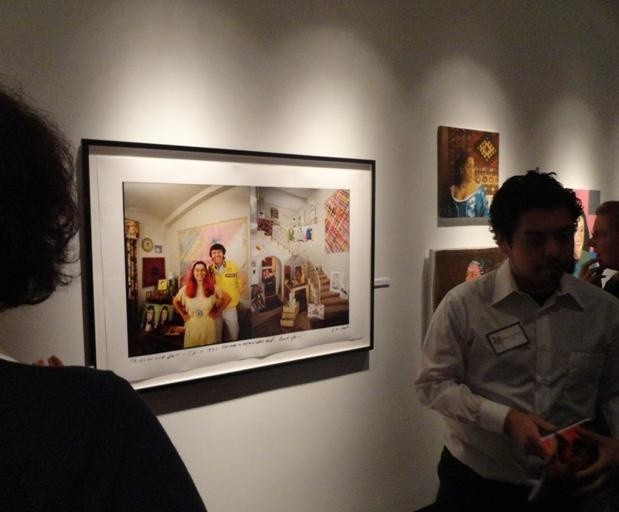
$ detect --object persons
[140,305,156,332]
[156,306,170,328]
[208,243,242,342]
[0,82,210,511]
[464,257,489,282]
[573,212,597,278]
[172,260,232,348]
[440,152,489,217]
[578,200,619,299]
[416,166,619,512]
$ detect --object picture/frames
[82,138,376,392]
